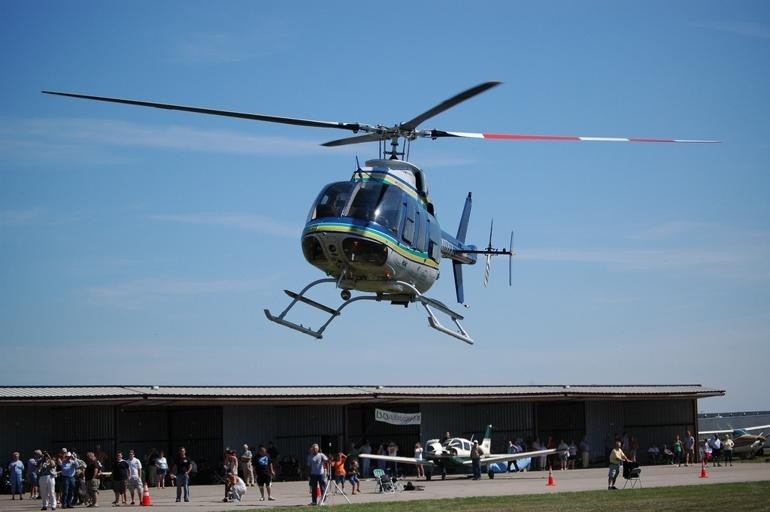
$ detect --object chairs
[373,469,405,494]
[623,462,643,488]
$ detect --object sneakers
[19,497,23,500]
[222,498,228,502]
[268,497,275,500]
[308,502,317,506]
[334,489,361,496]
[29,495,38,499]
[175,497,181,502]
[472,476,481,480]
[505,466,575,474]
[259,497,265,501]
[41,507,47,511]
[671,463,734,468]
[184,497,189,502]
[62,502,97,509]
[51,507,56,511]
[112,500,135,507]
[11,496,15,500]
[607,485,618,490]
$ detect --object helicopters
[38,81,727,349]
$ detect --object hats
[474,440,479,444]
[34,449,44,456]
[62,447,67,453]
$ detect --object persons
[8,441,299,510]
[332,442,400,494]
[507,431,733,471]
[306,443,330,505]
[608,440,632,489]
[471,439,484,480]
[414,442,425,478]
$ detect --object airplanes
[355,425,566,480]
[697,418,770,460]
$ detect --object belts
[609,463,619,466]
[63,476,74,479]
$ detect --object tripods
[318,458,352,507]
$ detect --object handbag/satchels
[258,456,268,465]
[49,468,57,477]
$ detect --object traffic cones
[698,460,710,479]
[316,480,323,497]
[546,465,558,486]
[138,481,154,508]
